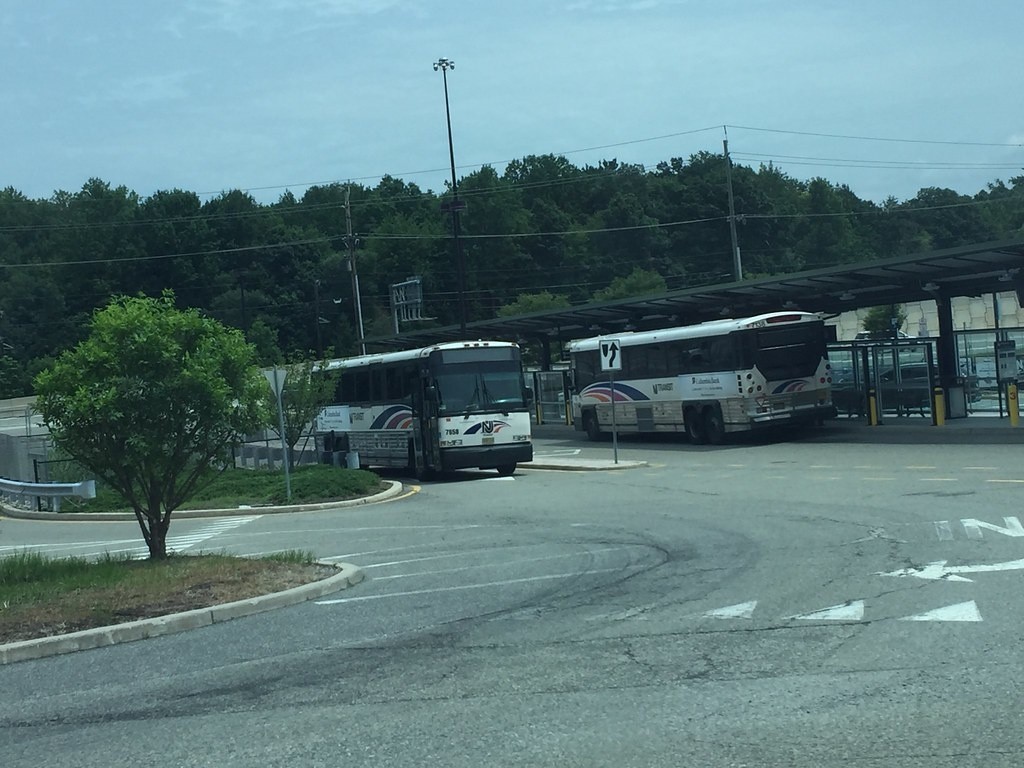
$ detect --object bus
[311,341,534,482]
[566,311,837,447]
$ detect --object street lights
[433,57,461,205]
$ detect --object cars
[869,362,982,409]
